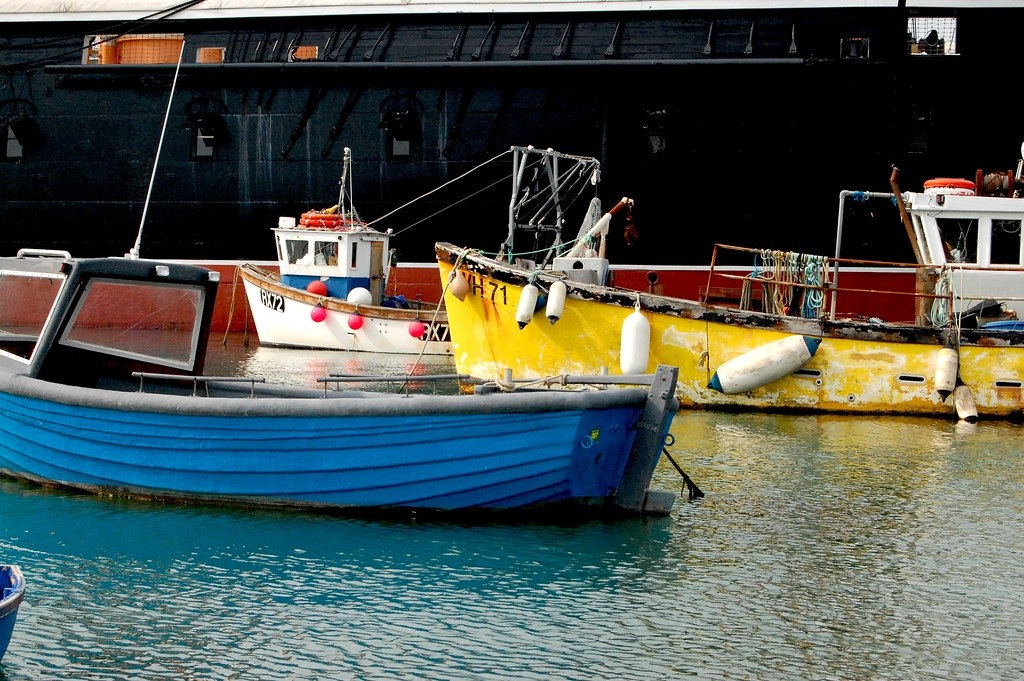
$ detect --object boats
[0,40,682,518]
[235,146,458,354]
[430,164,1023,421]
[0,562,27,663]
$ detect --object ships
[0,5,1023,266]
